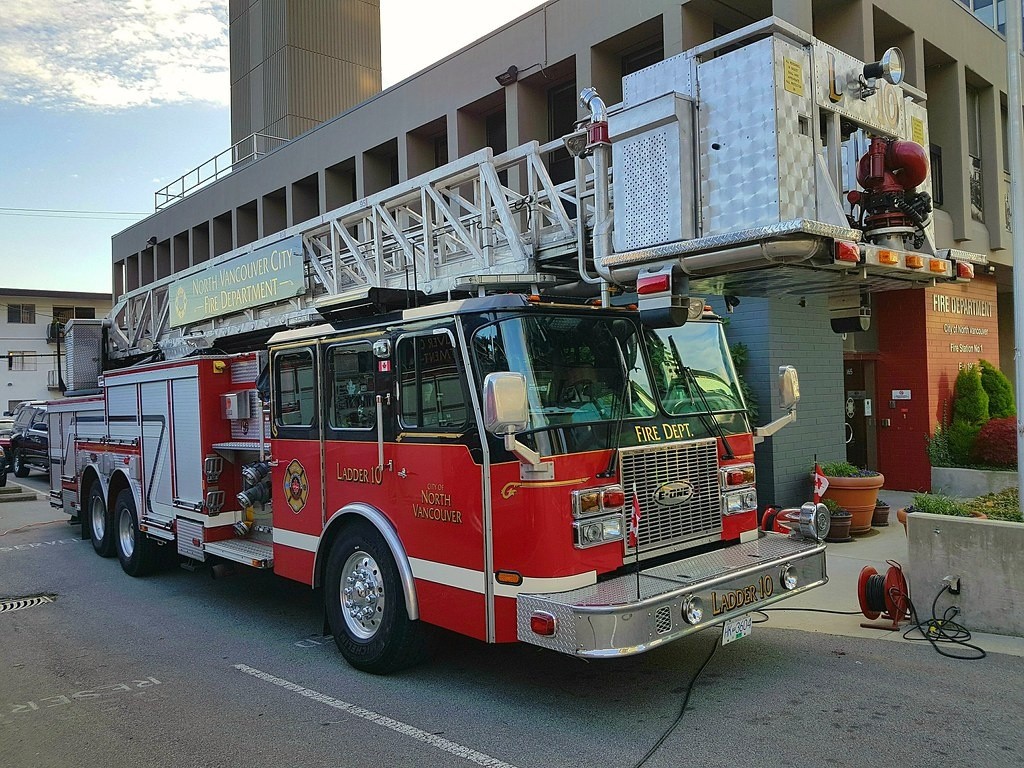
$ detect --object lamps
[724,294,739,309]
[857,47,906,100]
[494,65,518,87]
[146,236,157,245]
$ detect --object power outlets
[726,306,733,313]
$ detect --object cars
[0,400,46,487]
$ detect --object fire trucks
[47,13,974,687]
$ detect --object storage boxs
[220,389,250,422]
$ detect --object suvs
[12,401,49,479]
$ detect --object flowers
[851,469,879,478]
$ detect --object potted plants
[871,499,890,527]
[809,462,885,534]
[825,499,852,541]
[897,487,986,538]
